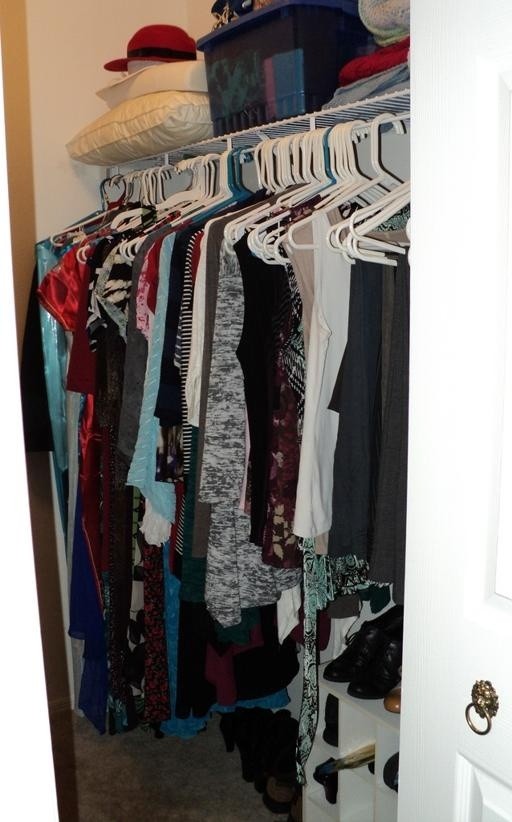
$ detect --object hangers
[45,115,410,273]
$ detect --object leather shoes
[324,604,403,713]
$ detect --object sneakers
[219,706,300,815]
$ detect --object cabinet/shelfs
[301,661,399,820]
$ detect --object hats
[103,25,199,73]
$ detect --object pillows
[67,90,213,165]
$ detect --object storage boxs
[194,2,369,133]
